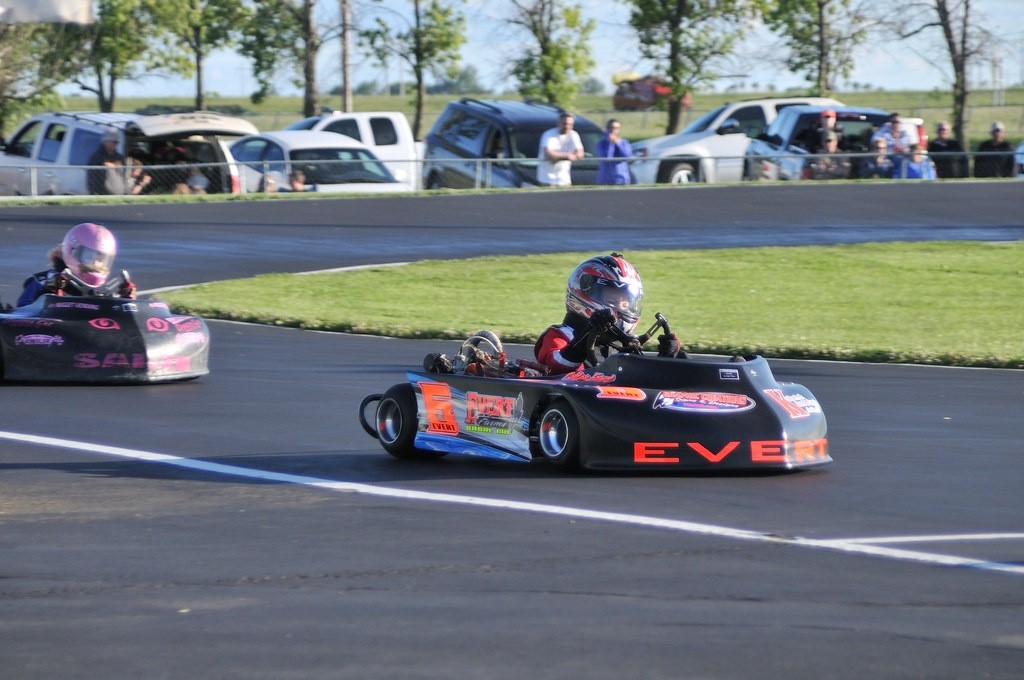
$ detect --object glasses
[891,120,902,124]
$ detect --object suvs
[0,103,260,197]
[630,96,847,184]
[741,104,892,183]
[422,97,607,189]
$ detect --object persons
[742,109,1019,182]
[537,112,584,188]
[15,223,135,309]
[534,252,682,377]
[595,118,634,185]
[85,132,310,197]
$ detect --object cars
[225,130,412,193]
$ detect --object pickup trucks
[280,105,426,190]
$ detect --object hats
[821,130,837,144]
[98,126,121,143]
[937,121,950,131]
[821,109,837,118]
[990,121,1003,132]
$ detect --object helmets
[61,223,117,289]
[564,252,643,336]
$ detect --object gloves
[587,307,616,332]
[44,270,71,295]
[117,281,137,299]
[657,333,682,359]
